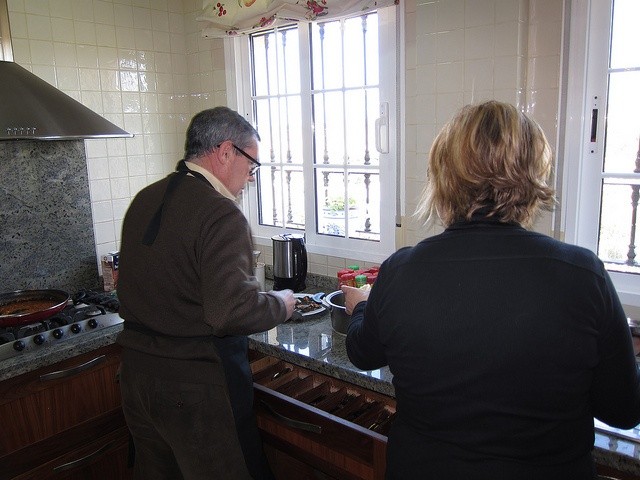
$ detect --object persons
[114,106,298,479]
[340,101,640,480]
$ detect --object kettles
[271,234,308,292]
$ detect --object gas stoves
[0,288,126,360]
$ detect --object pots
[0,288,70,324]
[326,289,360,337]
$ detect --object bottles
[254,250,266,295]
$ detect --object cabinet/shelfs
[0,340,136,479]
[591,464,639,480]
[247,347,397,479]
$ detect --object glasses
[217,144,261,175]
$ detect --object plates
[292,293,327,317]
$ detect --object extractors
[0,0,136,141]
